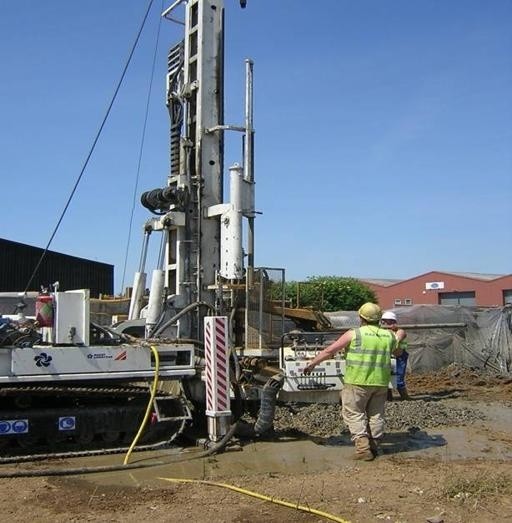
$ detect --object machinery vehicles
[0,0,398,463]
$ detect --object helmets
[358,302,382,322]
[380,311,397,323]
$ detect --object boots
[398,387,411,401]
[369,438,384,455]
[387,389,393,402]
[348,435,373,460]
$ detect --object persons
[301,301,400,461]
[380,310,409,401]
